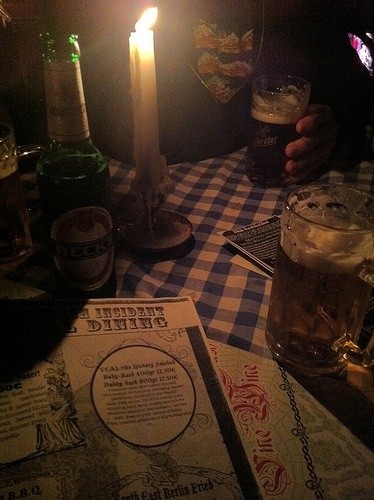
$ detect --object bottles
[36,32,118,308]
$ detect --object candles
[128,7,161,178]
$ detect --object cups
[245,73,310,187]
[264,185,374,376]
[0,125,33,274]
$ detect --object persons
[46,0,374,185]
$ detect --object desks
[0,146,374,451]
